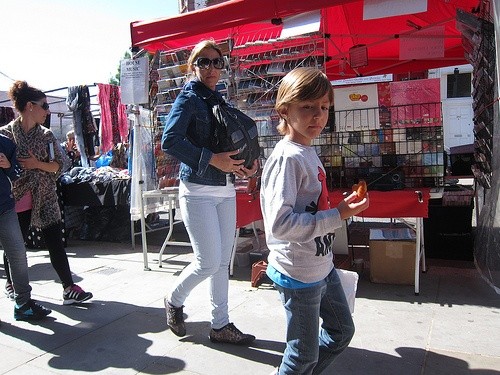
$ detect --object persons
[259,67,370,375]
[0,134,52,321]
[59,129,130,170]
[160,40,260,346]
[0,81,93,306]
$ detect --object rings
[231,169,233,172]
[23,164,24,167]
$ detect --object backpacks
[183,87,260,174]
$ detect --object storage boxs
[369,227,417,286]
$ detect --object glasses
[30,100,50,110]
[192,57,224,69]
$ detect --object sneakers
[4,281,16,301]
[209,323,255,345]
[163,295,187,337]
[62,284,93,304]
[13,299,52,319]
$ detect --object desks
[159,188,430,295]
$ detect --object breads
[352,180,367,203]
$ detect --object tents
[130,0,495,272]
